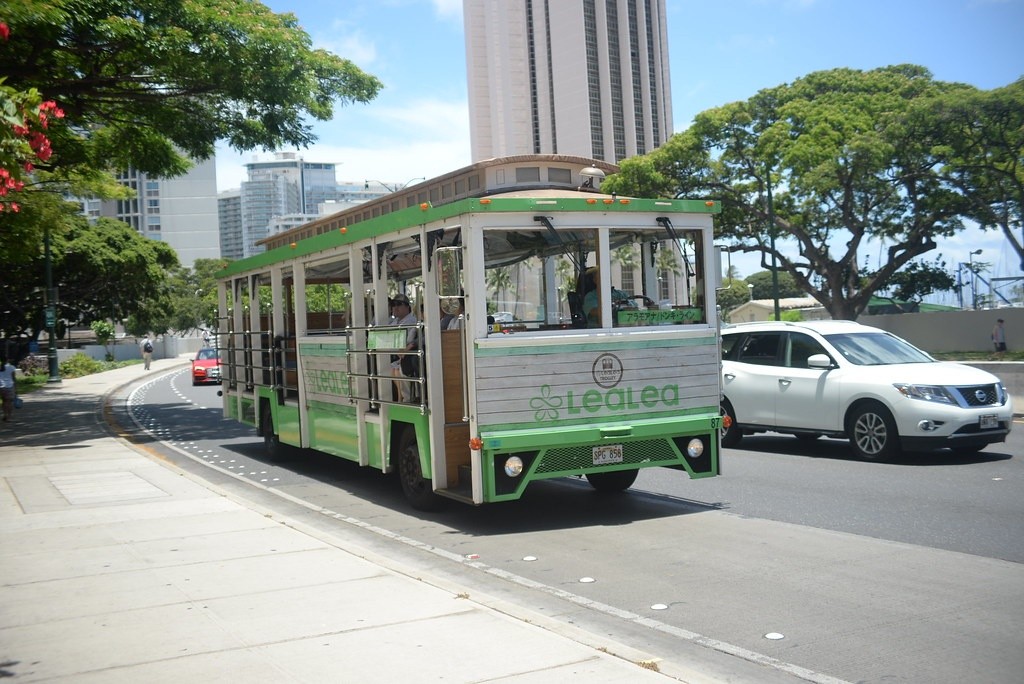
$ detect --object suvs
[721,319,1012,462]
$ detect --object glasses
[391,302,405,307]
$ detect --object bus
[212,153,731,511]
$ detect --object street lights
[970,249,982,309]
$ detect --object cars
[189,349,222,386]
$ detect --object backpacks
[144,339,153,353]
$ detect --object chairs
[567,267,596,329]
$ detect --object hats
[391,294,409,304]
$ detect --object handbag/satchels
[401,355,414,376]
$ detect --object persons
[367,289,465,404]
[140,335,152,369]
[202,328,210,348]
[584,268,637,327]
[990,319,1006,361]
[0,355,18,422]
